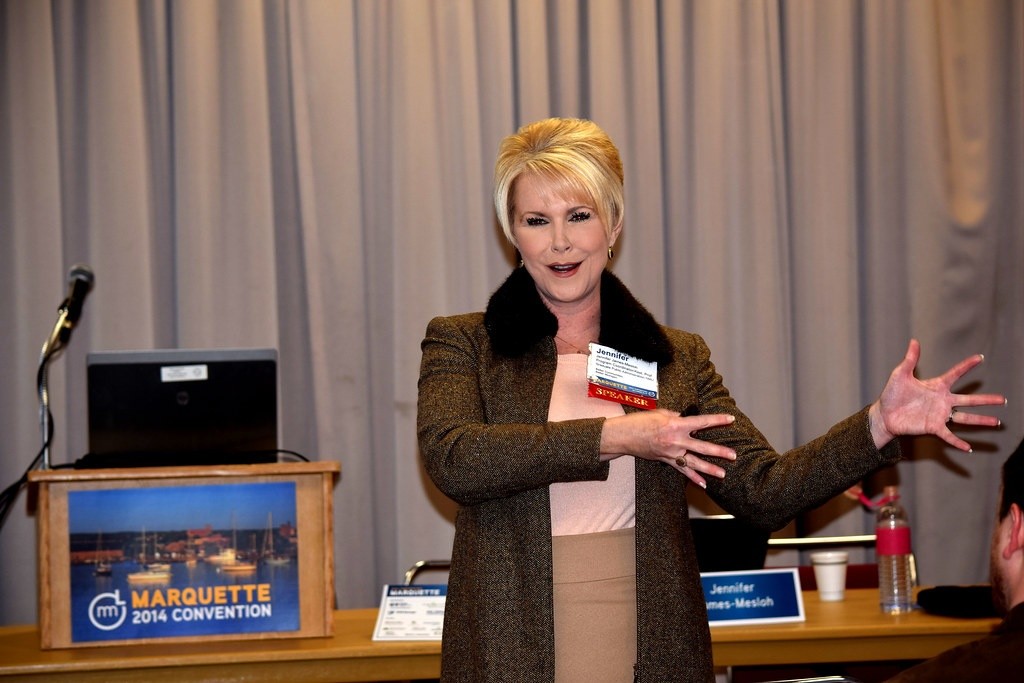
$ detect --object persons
[414,114,1008,683]
[876,436,1024,683]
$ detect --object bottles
[878,486,912,615]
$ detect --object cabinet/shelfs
[26,461,344,649]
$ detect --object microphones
[54,262,96,346]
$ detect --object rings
[676,450,689,467]
[949,408,959,423]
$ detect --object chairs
[405,515,767,587]
[768,535,913,589]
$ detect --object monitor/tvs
[83,347,279,465]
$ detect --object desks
[1,581,1005,682]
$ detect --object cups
[810,550,847,601]
